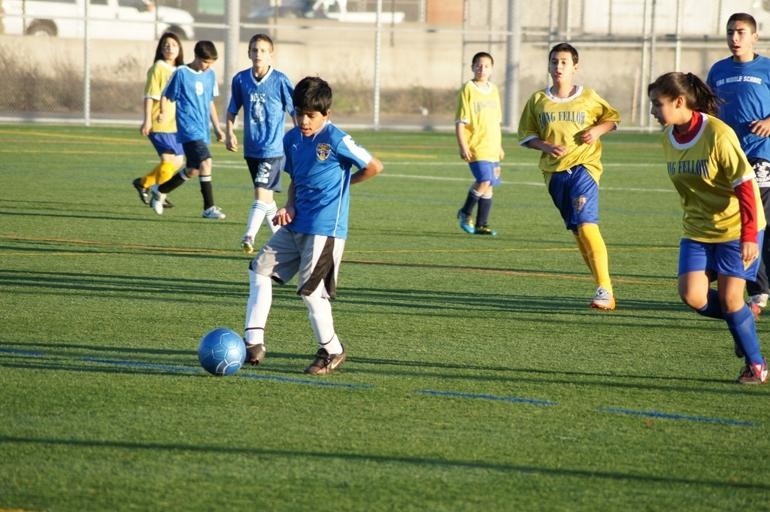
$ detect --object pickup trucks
[305,0,406,23]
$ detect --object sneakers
[150,184,166,216]
[240,235,255,254]
[202,206,225,219]
[747,294,769,313]
[163,199,174,208]
[241,337,266,365]
[735,302,759,358]
[737,357,769,385]
[456,208,474,234]
[132,178,149,204]
[304,343,346,377]
[474,224,496,236]
[591,286,616,311]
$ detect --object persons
[516,41,622,310]
[224,33,297,256]
[133,31,185,208]
[454,51,506,236]
[706,12,770,314]
[647,71,769,384]
[150,39,227,220]
[242,75,384,374]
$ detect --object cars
[254,5,302,22]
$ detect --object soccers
[197,327,247,377]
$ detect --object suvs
[1,0,197,42]
